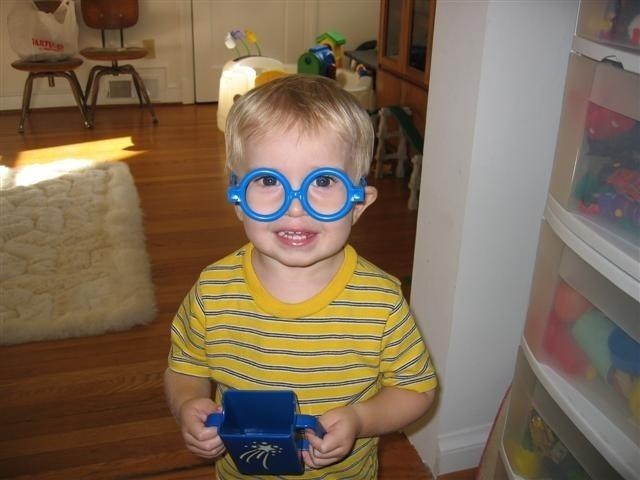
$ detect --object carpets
[0,158,160,345]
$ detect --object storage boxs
[478,346,623,480]
[540,45,639,285]
[517,203,640,478]
[573,0,638,73]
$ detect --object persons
[161,73,438,480]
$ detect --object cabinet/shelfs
[371,0,436,167]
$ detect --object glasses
[226,168,368,221]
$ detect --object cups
[204,389,325,476]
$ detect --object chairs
[9,1,160,135]
